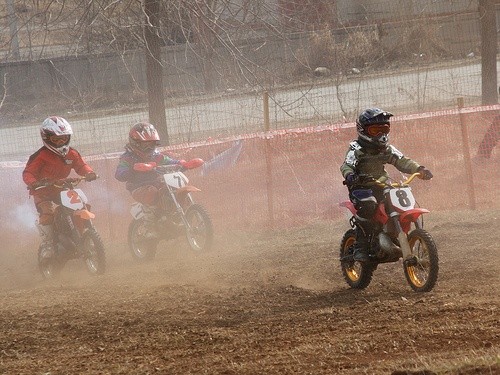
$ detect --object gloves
[418,166,433,180]
[85,171,96,182]
[347,173,360,188]
[31,181,43,191]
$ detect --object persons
[115,123,205,233]
[341,107,433,263]
[22,114,99,264]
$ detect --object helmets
[40,116,71,157]
[356,108,394,146]
[126,122,160,159]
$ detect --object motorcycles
[127,158,214,261]
[339,172,439,293]
[37,175,107,281]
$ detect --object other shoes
[145,231,160,239]
[354,248,369,261]
